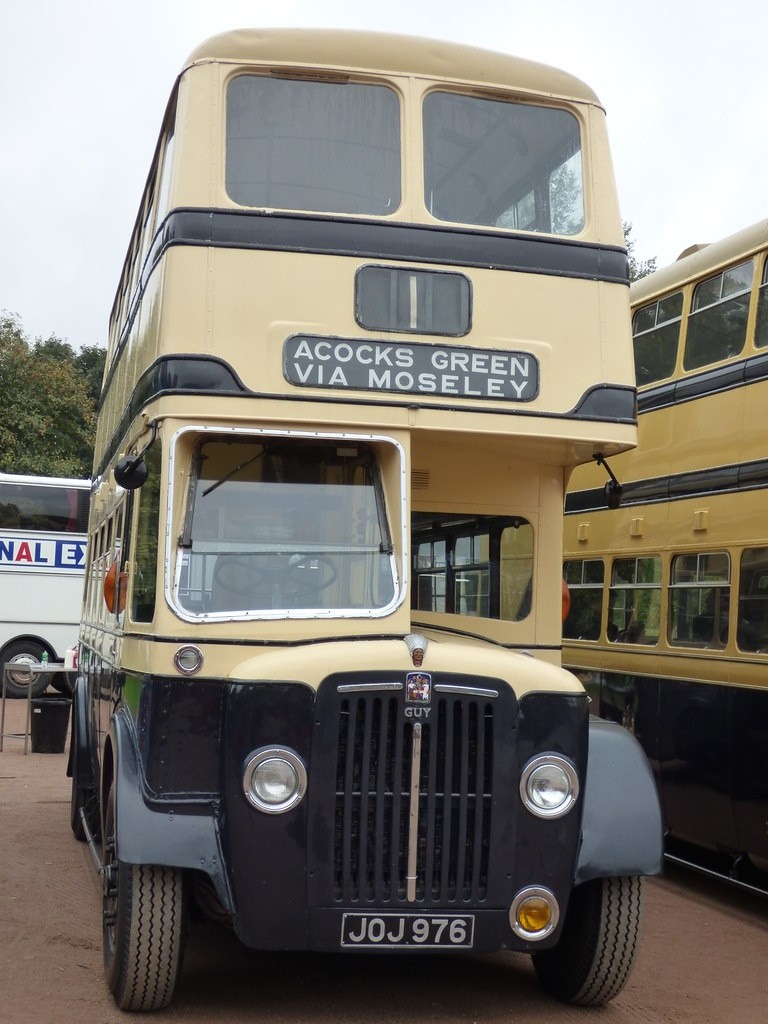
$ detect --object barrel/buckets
[31,698,71,753]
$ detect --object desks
[0,662,77,755]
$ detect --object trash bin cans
[31,698,72,753]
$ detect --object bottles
[42,651,48,666]
[73,649,78,668]
[41,658,47,669]
[65,647,73,669]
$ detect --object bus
[475,217,767,899]
[0,470,92,699]
[62,26,668,1013]
